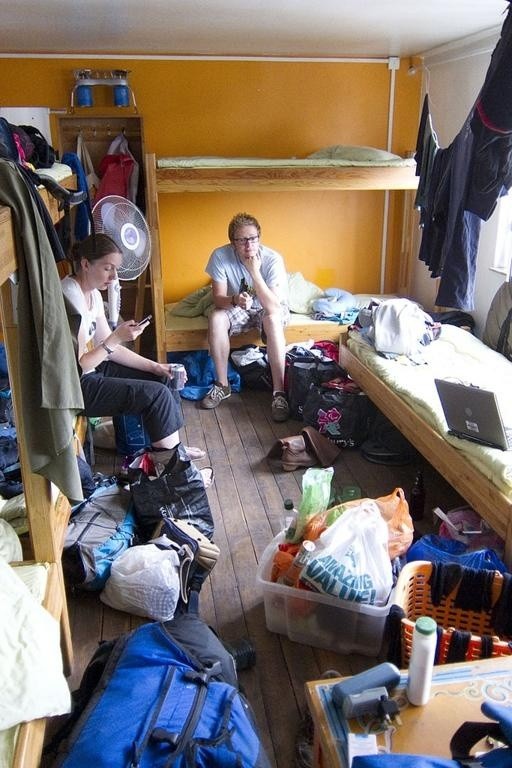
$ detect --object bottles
[112,70,129,107]
[271,540,317,588]
[408,468,426,521]
[75,70,93,107]
[404,615,439,709]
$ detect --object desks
[303,655,511,768]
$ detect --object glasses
[234,235,259,243]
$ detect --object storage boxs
[256,525,402,657]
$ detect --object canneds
[170,364,184,391]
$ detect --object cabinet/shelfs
[48,113,146,354]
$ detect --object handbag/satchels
[74,135,140,209]
[153,514,221,607]
[288,356,348,422]
[303,377,378,452]
[127,440,216,542]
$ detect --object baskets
[392,555,511,660]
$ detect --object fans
[88,194,152,450]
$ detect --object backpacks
[43,619,270,768]
[58,483,138,594]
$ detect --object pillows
[305,144,404,160]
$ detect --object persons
[58,233,212,482]
[201,212,298,422]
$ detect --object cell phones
[137,314,152,327]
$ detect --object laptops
[434,378,512,451]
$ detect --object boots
[301,426,342,466]
[267,436,316,472]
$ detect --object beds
[145,151,428,363]
[0,173,87,767]
[338,325,512,573]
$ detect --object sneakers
[201,381,231,409]
[200,467,214,489]
[272,390,289,422]
[181,440,205,461]
[296,670,342,767]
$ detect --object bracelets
[101,342,115,354]
[231,294,237,307]
